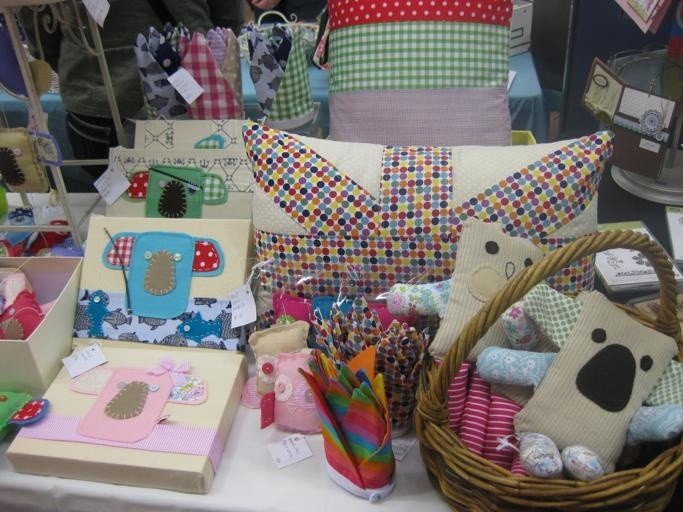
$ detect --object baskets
[413,229,683,512]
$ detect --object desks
[1,49,546,175]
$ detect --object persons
[244,0,295,24]
[18,0,216,179]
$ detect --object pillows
[241,120,616,345]
